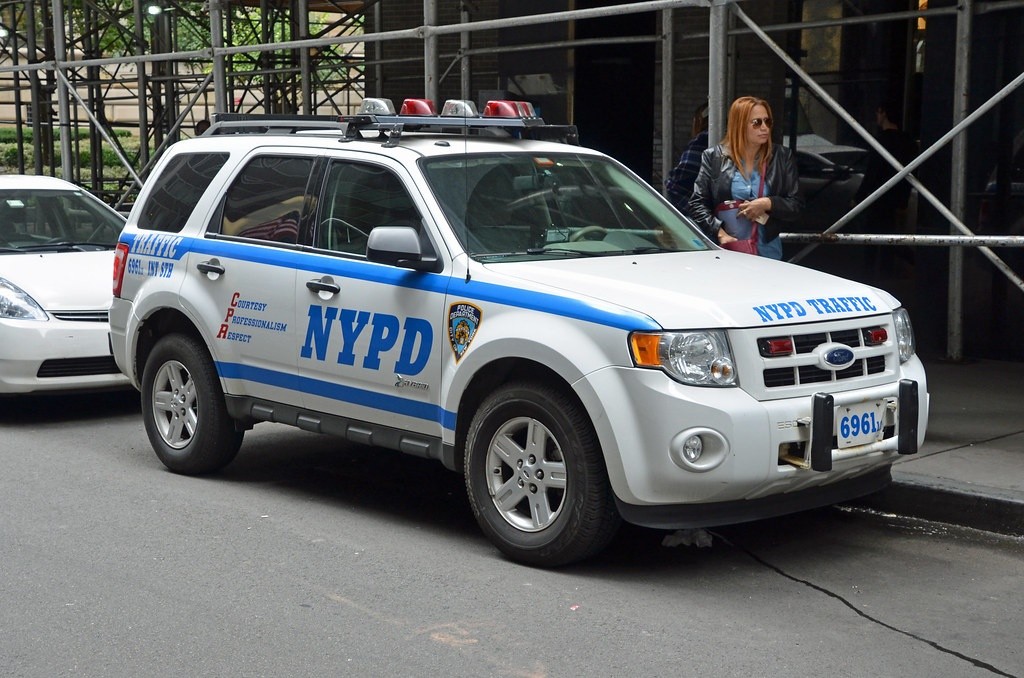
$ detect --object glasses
[748,118,772,129]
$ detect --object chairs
[470,191,534,253]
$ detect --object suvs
[103,86,931,566]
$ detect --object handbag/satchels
[714,199,758,255]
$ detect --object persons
[690,96,802,261]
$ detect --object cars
[0,171,137,413]
[787,143,922,241]
